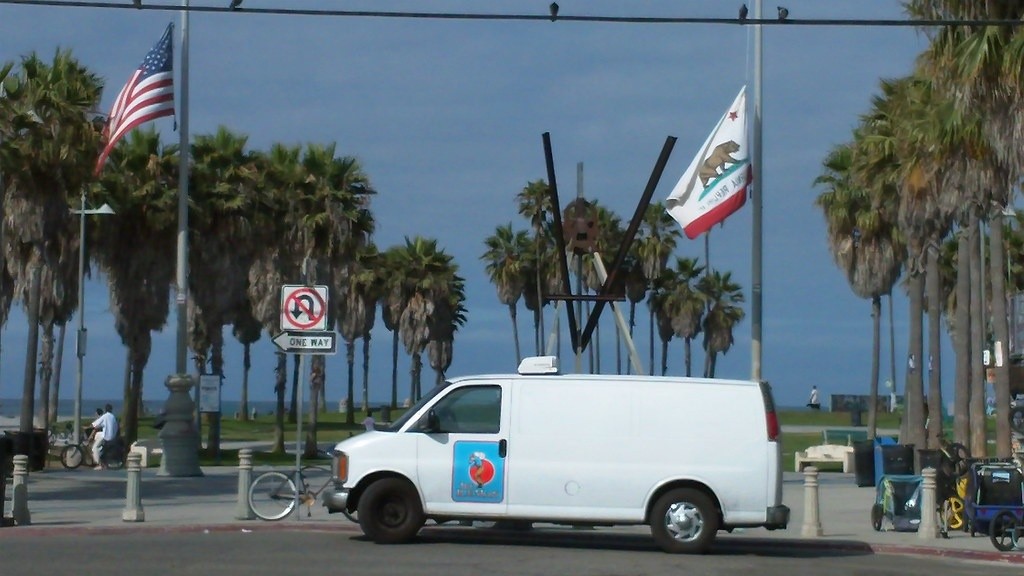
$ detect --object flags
[665,85,753,240]
[92,22,175,175]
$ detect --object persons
[80,404,118,471]
[810,385,818,404]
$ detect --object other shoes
[94,466,102,471]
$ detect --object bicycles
[247,448,333,521]
[59,436,127,470]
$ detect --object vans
[321,355,792,554]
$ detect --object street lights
[67,188,116,445]
